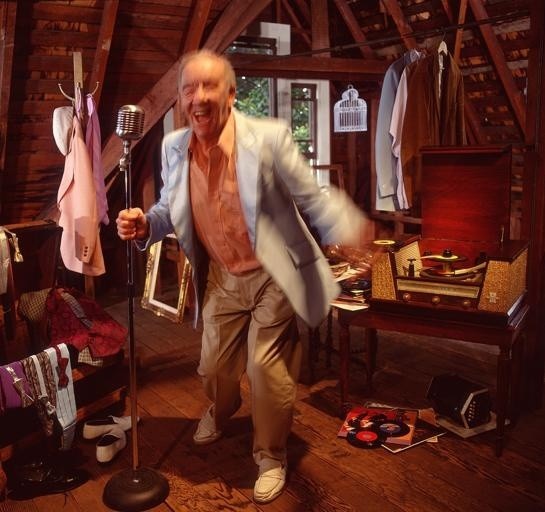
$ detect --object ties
[59,289,93,329]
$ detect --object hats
[53,105,73,157]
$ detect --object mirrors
[140,231,192,324]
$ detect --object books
[337,409,418,446]
[419,406,510,439]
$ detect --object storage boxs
[0,219,135,464]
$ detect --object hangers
[412,25,451,61]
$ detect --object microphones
[114,101,146,149]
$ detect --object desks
[333,288,532,459]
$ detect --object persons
[115,48,376,503]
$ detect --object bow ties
[58,358,68,387]
[40,395,57,416]
[13,379,33,409]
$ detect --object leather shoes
[83,416,140,443]
[194,396,242,444]
[254,452,288,503]
[96,429,127,465]
[5,451,88,500]
